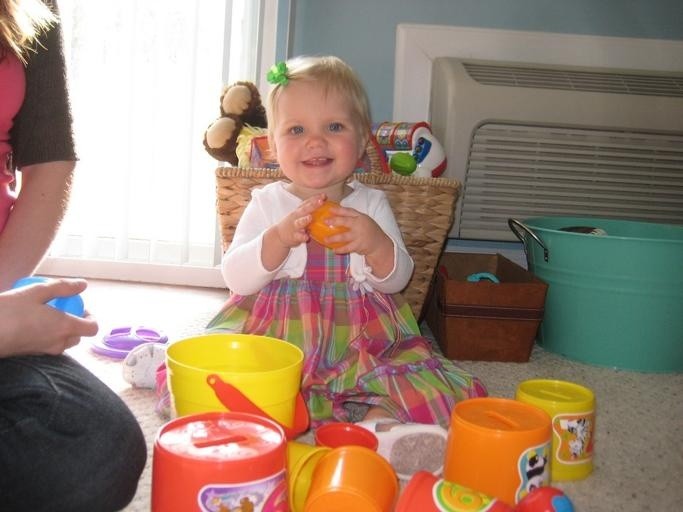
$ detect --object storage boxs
[429,251,548,362]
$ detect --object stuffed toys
[199,82,265,163]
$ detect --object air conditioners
[426,56,683,242]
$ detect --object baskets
[216,132,461,331]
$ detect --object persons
[160,57,492,431]
[0,0,147,511]
